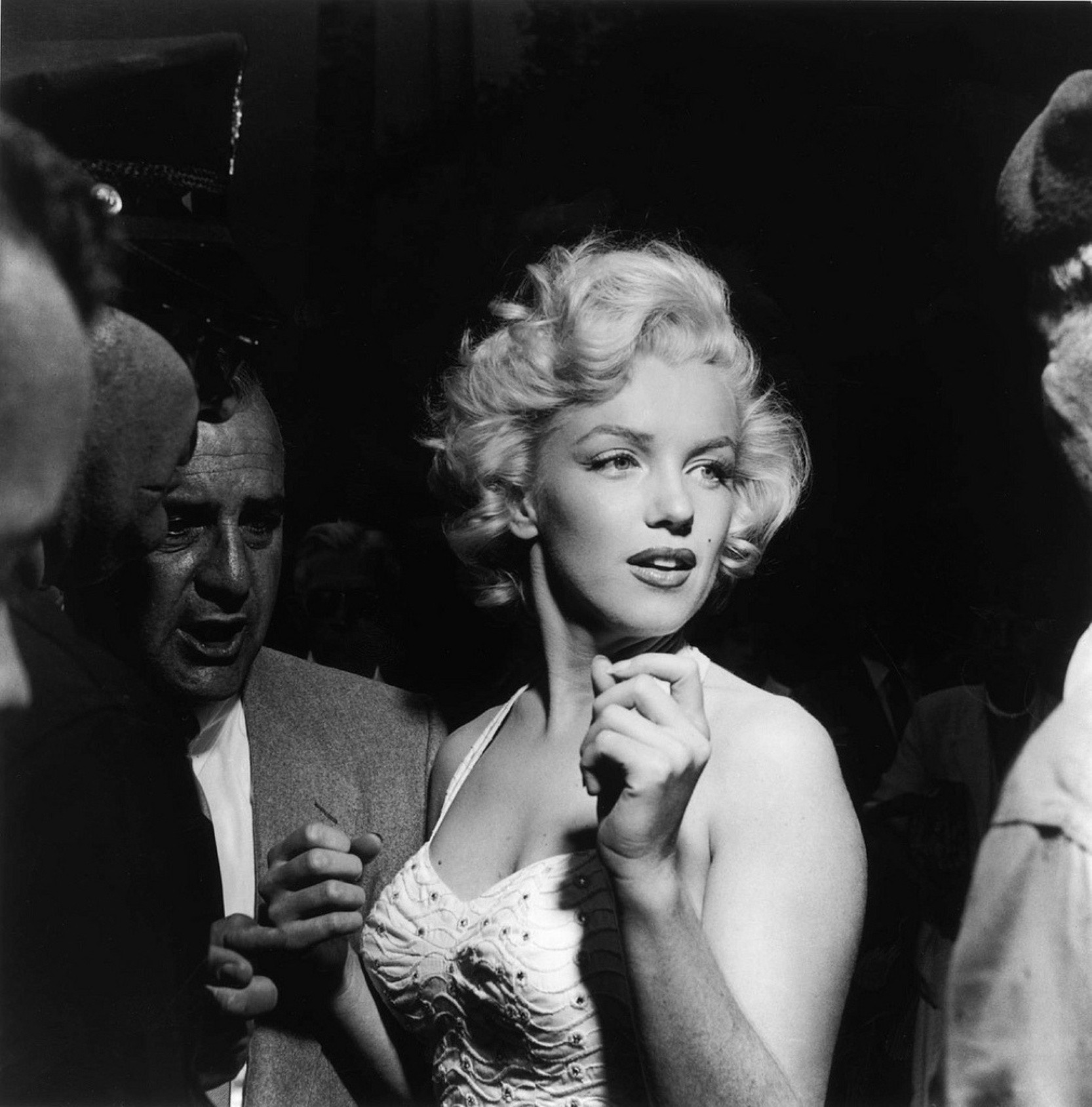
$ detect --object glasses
[87,278,278,401]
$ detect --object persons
[265,229,874,1106]
[920,68,1088,1107]
[3,116,449,1104]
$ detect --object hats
[1,27,258,255]
